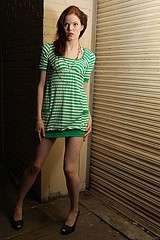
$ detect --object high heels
[13,204,24,231]
[61,208,81,235]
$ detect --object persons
[12,5,96,234]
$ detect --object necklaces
[62,46,81,69]
